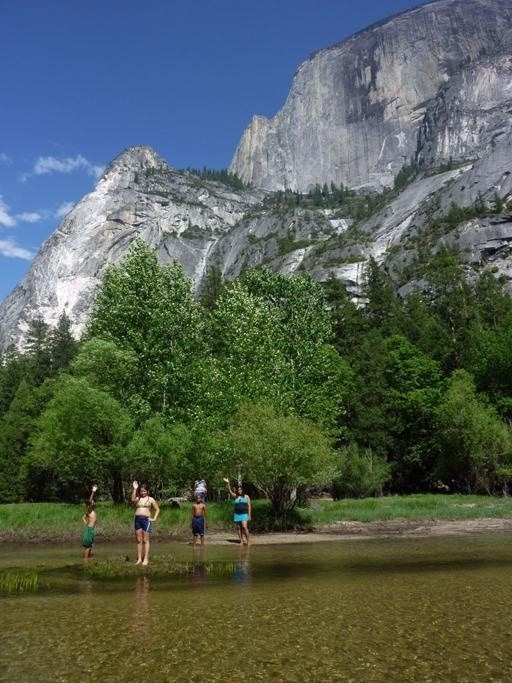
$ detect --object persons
[130,480,160,566]
[135,575,150,609]
[190,546,205,593]
[168,498,180,511]
[223,477,251,545]
[232,545,251,597]
[190,495,209,549]
[81,484,98,560]
[193,475,207,504]
